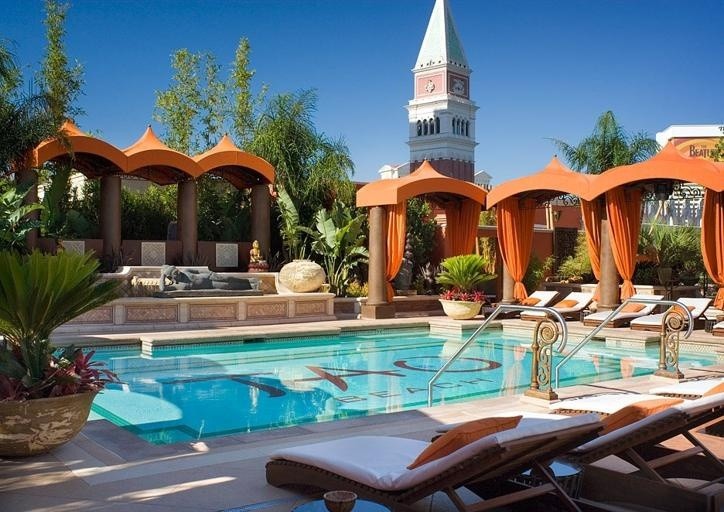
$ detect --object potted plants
[0,245,126,456]
[435,253,496,319]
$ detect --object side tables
[291,499,390,512]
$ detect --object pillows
[554,300,579,308]
[406,415,523,470]
[598,398,683,434]
[704,381,724,395]
[670,306,695,314]
[619,303,646,313]
[521,297,541,306]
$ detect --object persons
[250,239,264,263]
[159,265,252,290]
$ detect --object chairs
[647,378,724,399]
[519,291,594,322]
[264,411,605,512]
[584,293,664,328]
[497,290,561,318]
[431,391,724,511]
[629,297,713,332]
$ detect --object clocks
[452,82,465,95]
